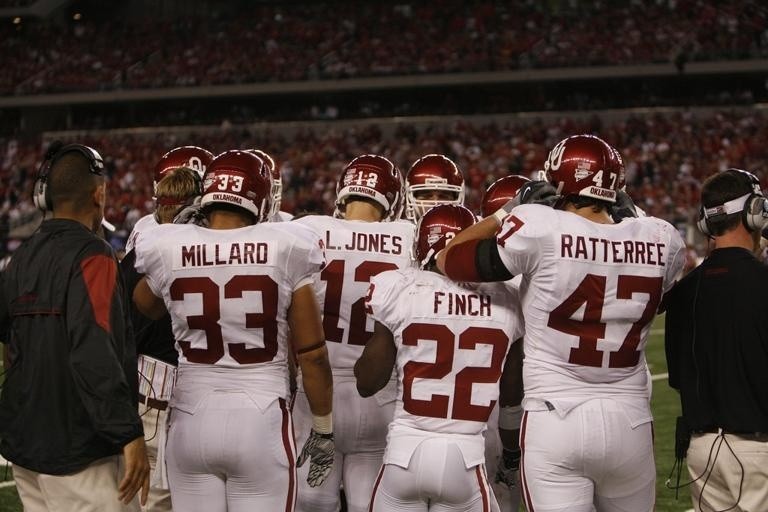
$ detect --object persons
[1,132,768,512]
[0,2,768,272]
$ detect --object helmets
[481,174,531,217]
[154,144,282,224]
[404,155,479,271]
[335,154,407,224]
[540,135,626,208]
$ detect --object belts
[138,394,167,410]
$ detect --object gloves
[296,411,335,489]
[493,447,522,492]
[496,181,565,226]
[610,190,641,225]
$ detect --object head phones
[697,168,768,236]
[32,144,104,212]
